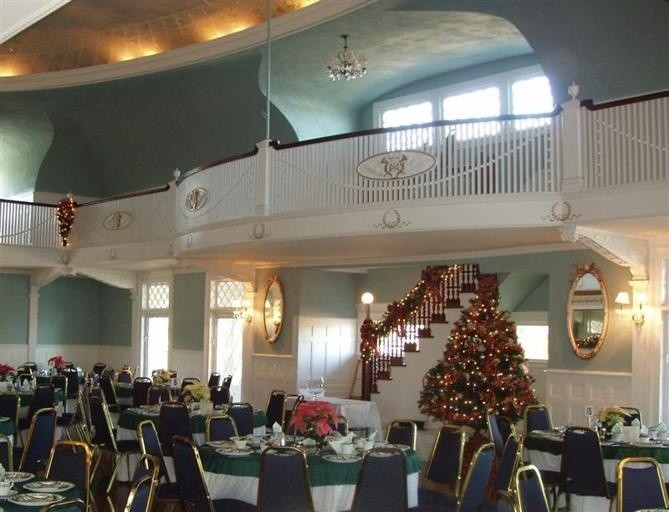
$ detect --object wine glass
[305,375,328,402]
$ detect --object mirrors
[263,276,285,344]
[567,262,609,359]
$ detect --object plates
[207,431,411,464]
[23,480,75,493]
[0,490,16,499]
[7,492,64,506]
[532,431,548,435]
[140,400,224,416]
[3,471,34,483]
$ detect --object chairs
[0,361,669,512]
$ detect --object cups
[612,422,669,445]
[0,480,14,496]
[0,382,16,392]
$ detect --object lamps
[361,292,374,320]
[326,34,369,82]
[232,300,252,323]
[616,293,648,327]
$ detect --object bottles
[16,376,21,392]
[594,422,606,439]
[40,368,48,377]
[23,378,30,392]
[0,463,5,482]
[49,365,53,375]
[30,378,35,391]
